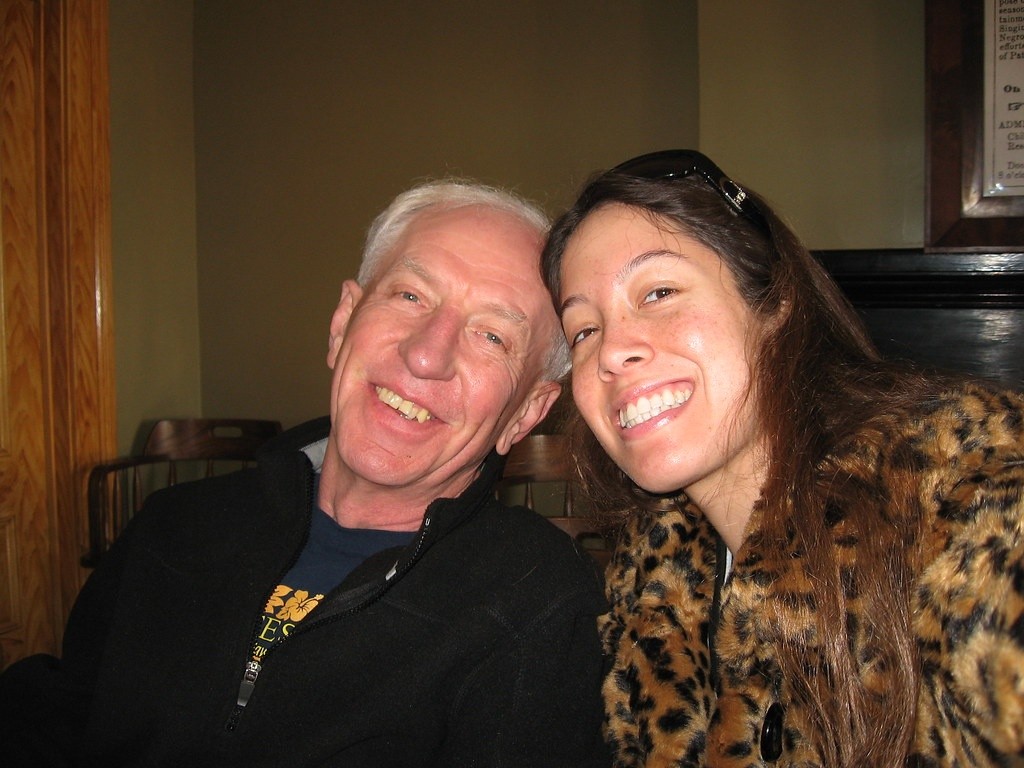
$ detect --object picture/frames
[924,0,1024,252]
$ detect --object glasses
[594,149,781,262]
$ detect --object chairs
[87,420,282,566]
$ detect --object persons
[0,177,602,768]
[538,149,1024,768]
